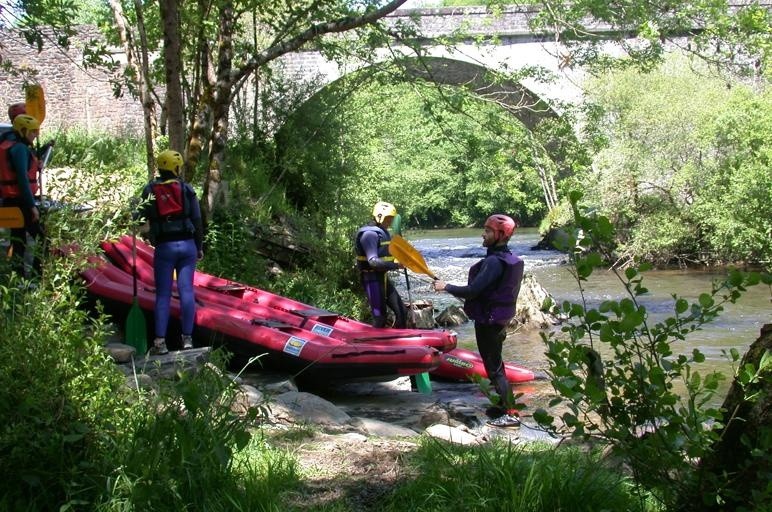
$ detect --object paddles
[389,214,432,395]
[125,226,147,356]
[388,235,466,304]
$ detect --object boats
[428,347,534,383]
[77,256,441,383]
[98,235,459,354]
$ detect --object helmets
[13,114,41,138]
[484,213,516,239]
[371,202,398,224]
[6,103,28,123]
[155,150,186,177]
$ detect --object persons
[353,201,408,329]
[0,101,56,164]
[430,215,525,427]
[0,114,48,294]
[134,149,208,356]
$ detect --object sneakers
[148,338,170,356]
[489,412,521,429]
[181,334,195,350]
